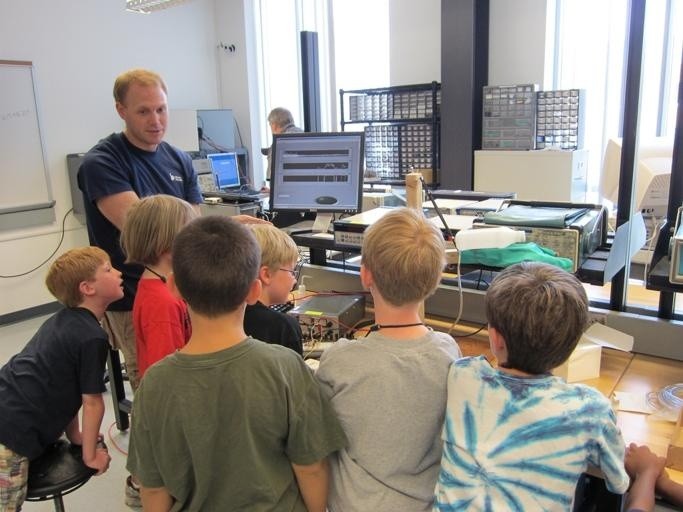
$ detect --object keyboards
[267,301,293,314]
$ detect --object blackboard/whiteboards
[0,58,57,215]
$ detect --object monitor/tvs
[268,132,365,240]
[206,154,244,194]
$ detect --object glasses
[279,268,299,279]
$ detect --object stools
[107,348,134,430]
[25,439,107,512]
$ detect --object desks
[286,289,681,499]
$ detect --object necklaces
[143,265,163,280]
[363,321,424,337]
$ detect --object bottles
[454,226,528,253]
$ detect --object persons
[261,107,315,229]
[619,442,683,512]
[314,209,462,511]
[118,193,197,378]
[125,215,349,512]
[0,245,125,512]
[430,260,632,511]
[77,68,204,394]
[244,223,303,354]
